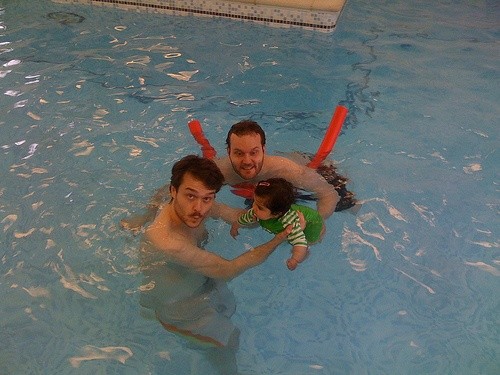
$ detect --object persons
[120,121,362,242]
[230,178,323,271]
[141,155,306,375]
